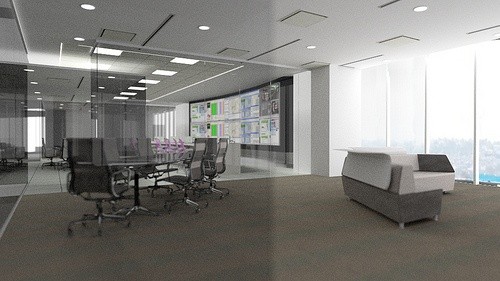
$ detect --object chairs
[41,134,229,236]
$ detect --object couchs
[340,148,455,228]
